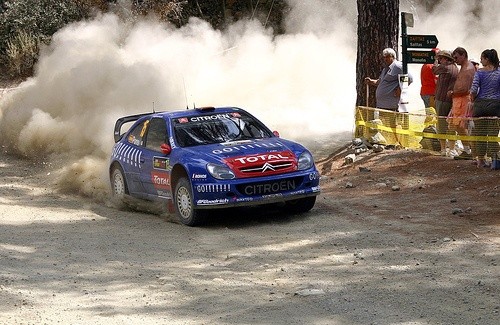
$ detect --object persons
[420,47,500,171]
[365,48,403,128]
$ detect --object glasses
[454,55,463,60]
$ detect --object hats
[434,50,454,61]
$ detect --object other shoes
[459,150,473,158]
[491,160,498,169]
[478,161,488,167]
[448,150,458,158]
[440,149,447,156]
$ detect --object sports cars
[109,105,322,226]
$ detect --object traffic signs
[406,35,439,48]
[406,50,436,64]
